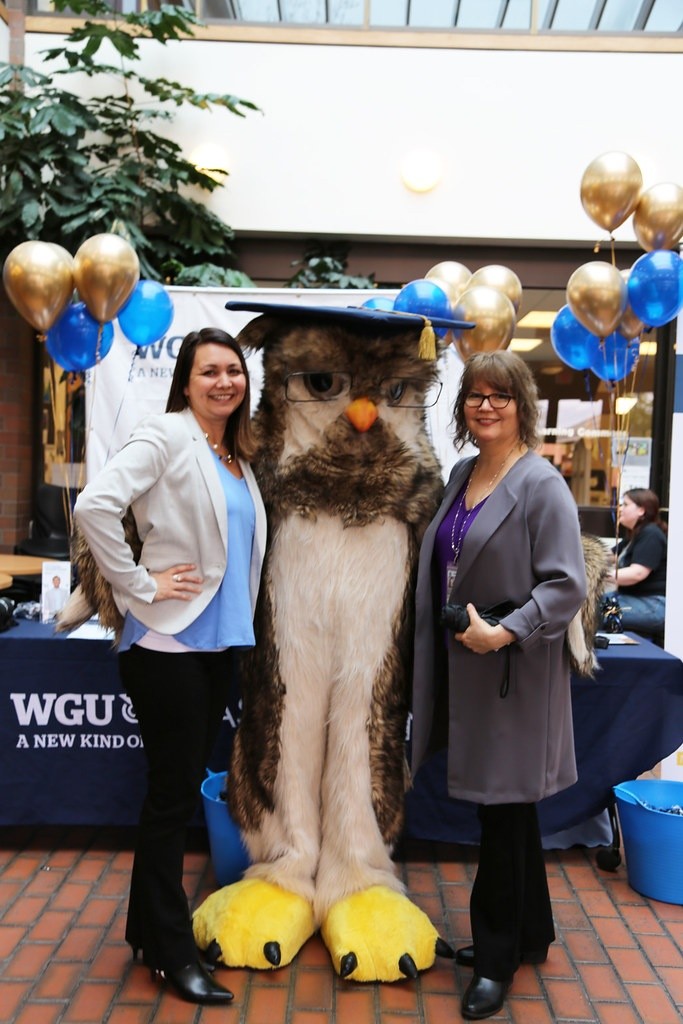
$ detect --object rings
[173,573,182,583]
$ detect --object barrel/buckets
[614,778,683,907]
[200,769,252,890]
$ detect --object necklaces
[450,440,520,553]
[205,432,233,464]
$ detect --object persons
[73,328,268,1004]
[598,488,667,628]
[408,351,589,1019]
[46,576,68,621]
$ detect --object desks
[0,606,683,871]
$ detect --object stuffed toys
[54,299,616,985]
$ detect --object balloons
[2,232,174,371]
[362,261,523,363]
[551,150,683,385]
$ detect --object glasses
[465,392,516,410]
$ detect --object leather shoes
[461,972,513,1021]
[456,944,474,968]
[131,938,235,1004]
